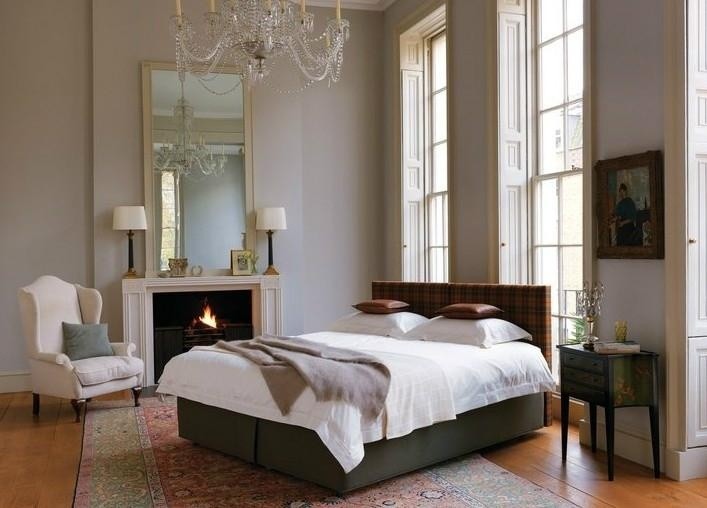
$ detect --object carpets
[71,391,579,508]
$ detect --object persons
[611,181,639,245]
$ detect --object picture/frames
[592,148,664,262]
[228,247,254,277]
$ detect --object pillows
[60,319,116,362]
[333,293,534,351]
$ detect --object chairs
[15,272,147,425]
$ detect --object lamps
[109,203,150,280]
[254,202,288,277]
[150,83,230,186]
[164,0,351,98]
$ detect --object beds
[155,279,553,496]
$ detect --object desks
[553,340,662,483]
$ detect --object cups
[615,319,627,342]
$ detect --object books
[591,339,642,354]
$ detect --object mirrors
[138,58,257,278]
